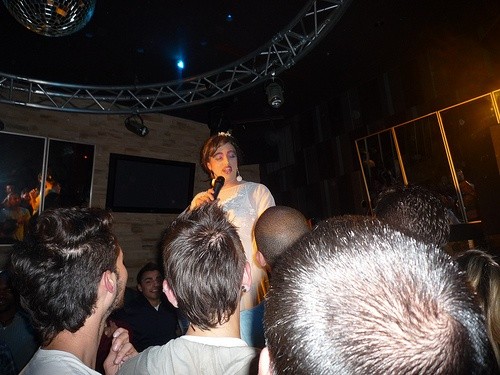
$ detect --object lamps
[124,120,149,137]
[266,83,284,107]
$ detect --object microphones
[212,176,225,200]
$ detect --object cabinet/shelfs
[0,131,97,247]
[354,88,500,240]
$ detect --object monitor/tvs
[106,153,196,214]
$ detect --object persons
[239,205,312,349]
[114,262,181,356]
[95,314,134,375]
[0,270,40,375]
[115,198,265,375]
[452,249,500,370]
[180,131,276,314]
[17,205,139,375]
[368,187,453,252]
[258,213,491,375]
[0,164,81,241]
